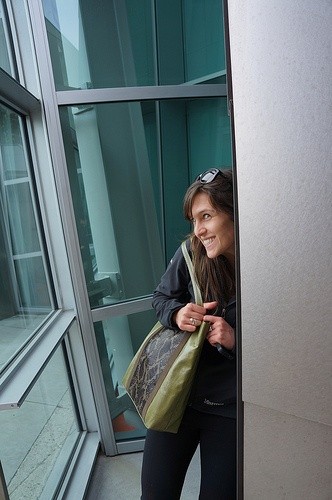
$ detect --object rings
[191,318,197,325]
[209,326,213,331]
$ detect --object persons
[141,168,237,500]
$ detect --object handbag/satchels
[121,239,212,432]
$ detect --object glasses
[193,168,232,184]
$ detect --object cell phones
[216,343,234,360]
